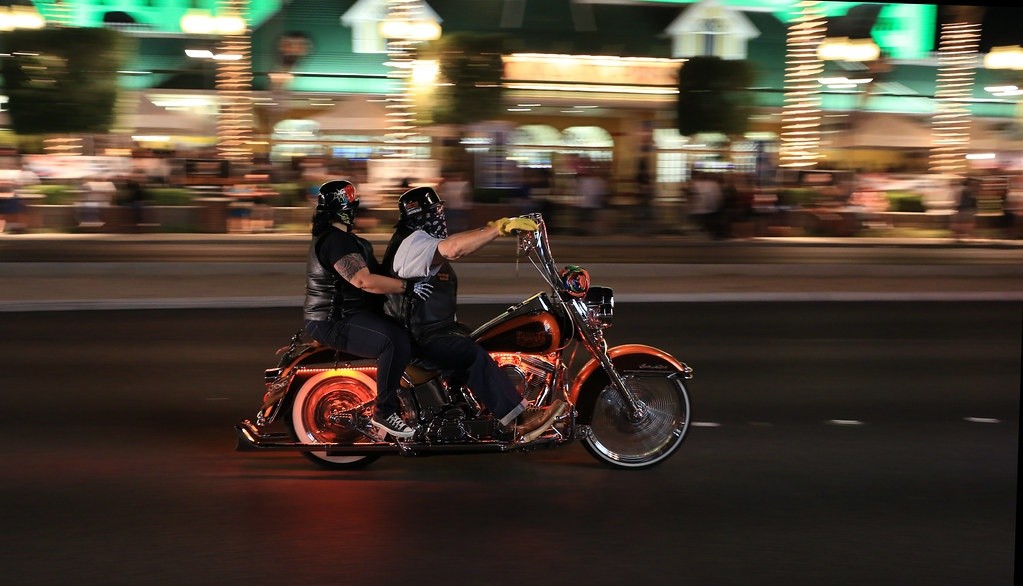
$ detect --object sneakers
[370,412,414,437]
[516,399,566,443]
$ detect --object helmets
[316,181,359,210]
[398,187,446,217]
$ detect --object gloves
[487,217,539,237]
[403,280,434,302]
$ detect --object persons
[296,179,435,437]
[376,187,564,442]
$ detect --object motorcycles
[232,211,694,473]
